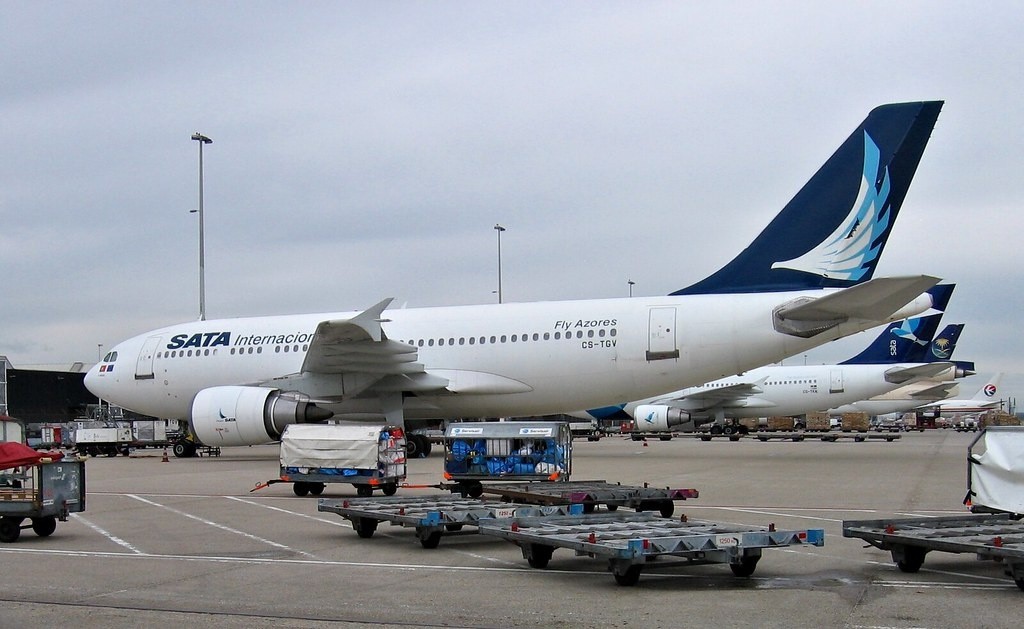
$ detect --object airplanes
[81,98,956,461]
[570,282,1009,440]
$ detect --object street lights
[628,279,635,298]
[189,132,214,320]
[494,224,505,304]
